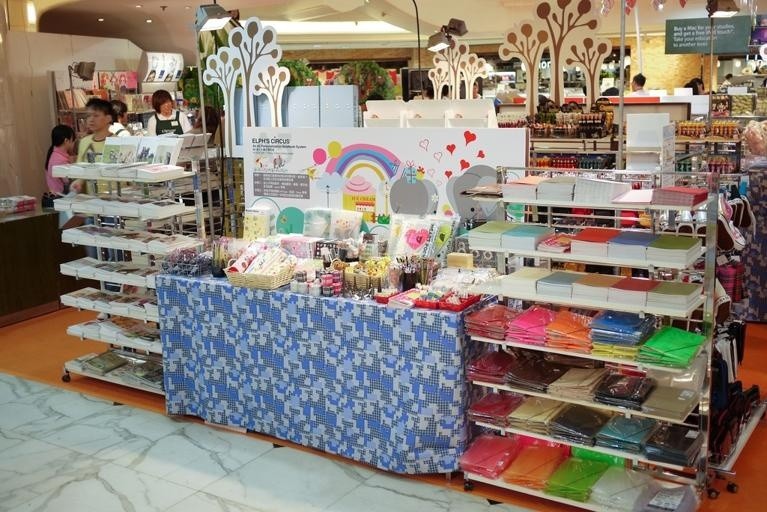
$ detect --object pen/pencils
[395,256,433,285]
[212,242,229,276]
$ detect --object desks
[155,244,492,484]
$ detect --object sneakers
[716,195,752,251]
[676,210,694,238]
[658,214,677,233]
[695,206,707,259]
[713,277,732,325]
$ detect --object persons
[44,98,131,320]
[684,78,716,95]
[628,73,650,96]
[148,90,194,136]
[473,81,482,99]
[720,74,733,89]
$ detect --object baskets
[330,258,390,291]
[223,258,295,289]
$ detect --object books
[53,192,197,220]
[59,256,161,289]
[60,286,160,322]
[61,224,199,254]
[53,133,212,181]
[65,319,162,353]
[65,352,164,391]
[469,177,708,313]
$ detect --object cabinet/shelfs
[51,104,245,398]
[462,103,764,512]
